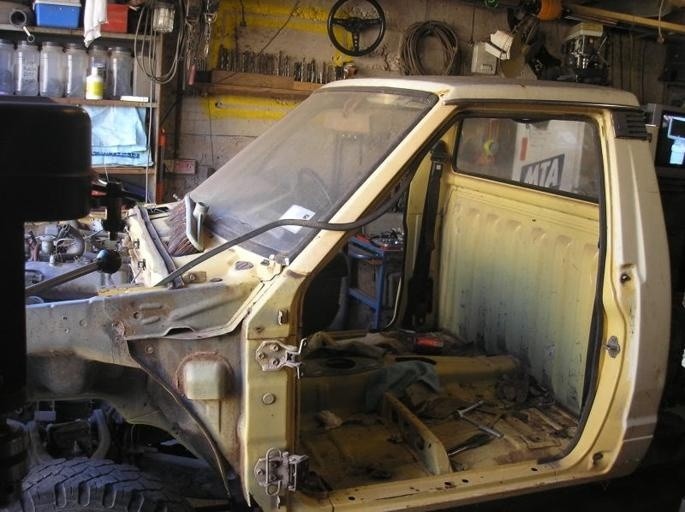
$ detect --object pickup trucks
[18,78,672,512]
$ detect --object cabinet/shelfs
[0,23,167,204]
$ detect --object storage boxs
[32,0,82,28]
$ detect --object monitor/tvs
[647,103,684,180]
